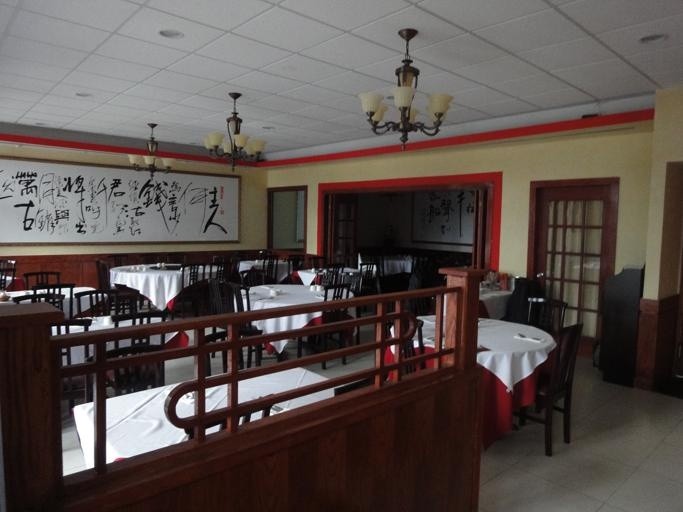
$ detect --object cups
[487,272,496,291]
[309,285,323,292]
[129,266,145,272]
[23,292,29,300]
[156,262,164,268]
[311,268,315,274]
[271,289,280,297]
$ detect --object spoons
[517,332,540,341]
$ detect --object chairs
[0,250,586,470]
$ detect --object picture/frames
[409,190,475,247]
[0,154,242,245]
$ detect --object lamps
[202,91,267,172]
[125,122,176,180]
[357,28,456,151]
